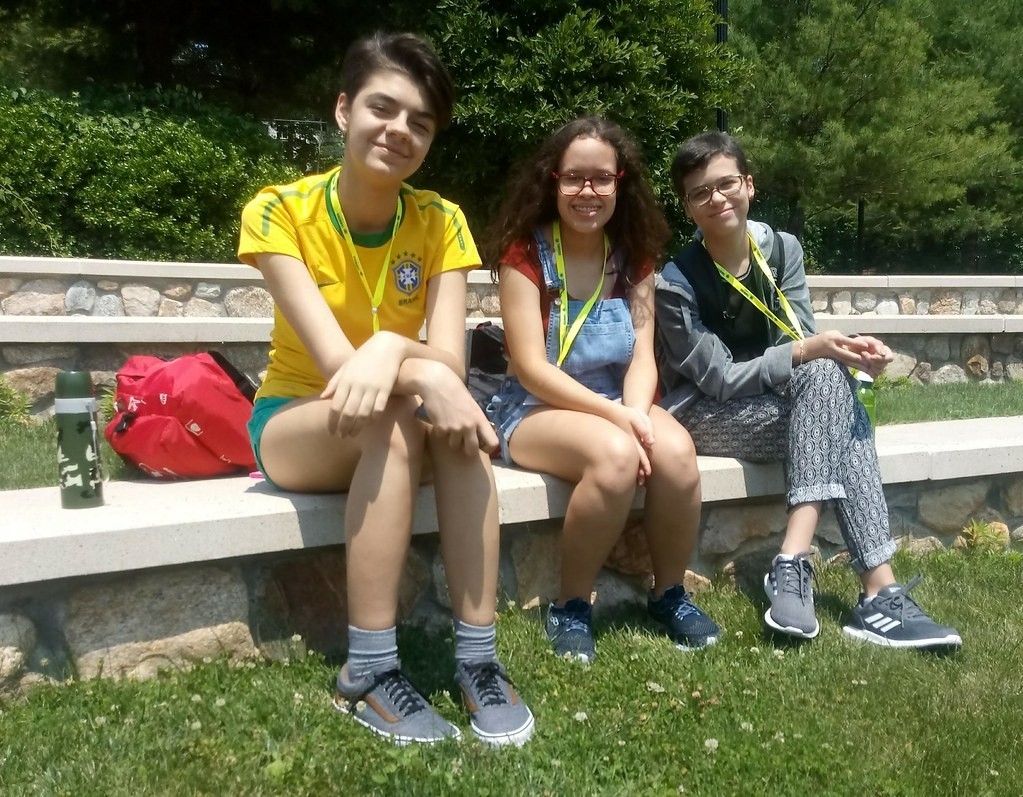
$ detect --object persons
[234,31,535,748]
[481,113,721,665]
[654,134,963,652]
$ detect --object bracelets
[799,340,805,364]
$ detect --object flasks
[54,370,105,510]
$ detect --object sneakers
[646,583,720,651]
[453,661,536,747]
[546,598,595,663]
[332,661,462,744]
[764,550,820,638]
[843,583,963,651]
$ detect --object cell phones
[414,402,498,448]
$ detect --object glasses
[683,175,747,208]
[552,172,625,196]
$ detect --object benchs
[0,413,1023,681]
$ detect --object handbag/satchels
[102,349,259,479]
